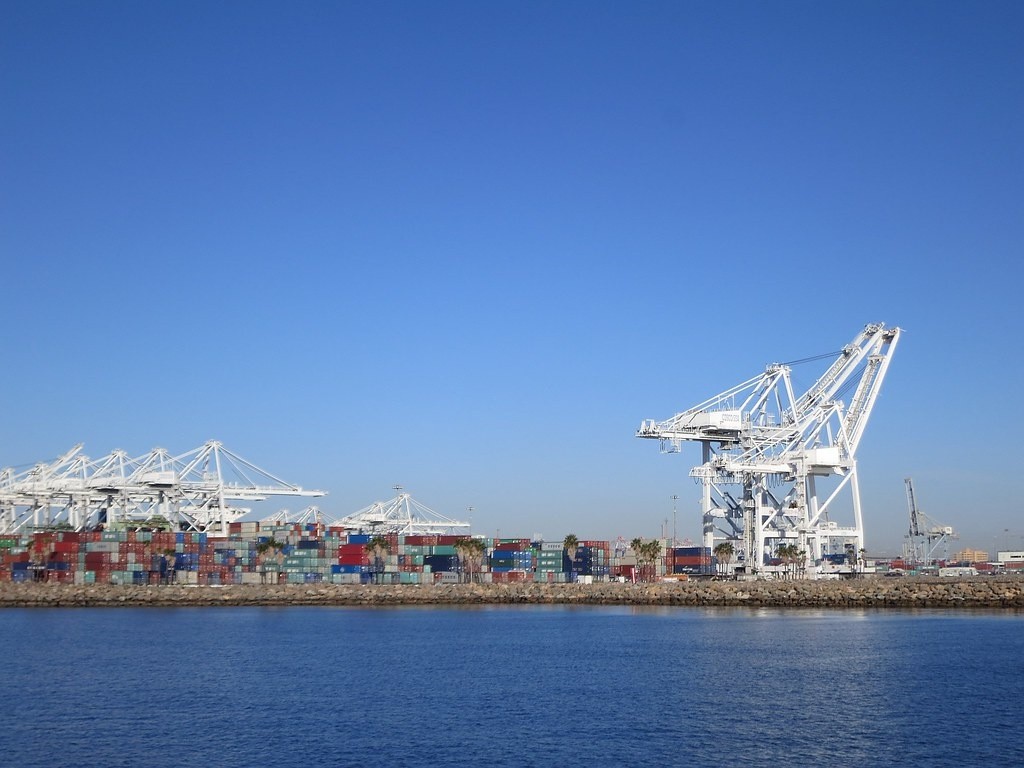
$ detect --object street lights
[392,485,403,534]
[468,507,475,524]
[671,495,679,574]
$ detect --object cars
[945,572,954,576]
[884,572,902,577]
[889,568,904,573]
